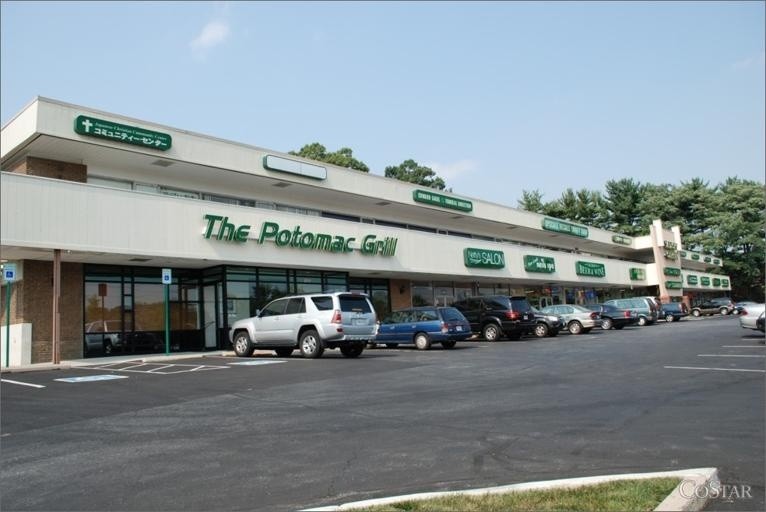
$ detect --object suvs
[447,295,536,339]
[228,289,377,358]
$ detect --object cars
[83,320,154,355]
[531,303,637,337]
[732,297,765,334]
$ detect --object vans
[604,296,687,325]
[378,306,473,350]
[691,297,736,317]
